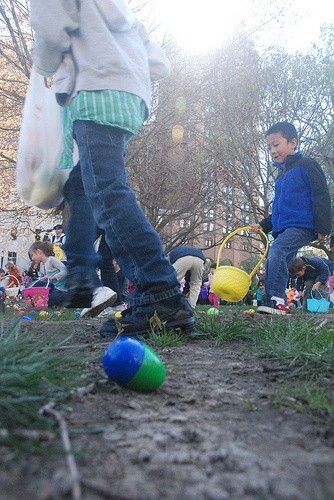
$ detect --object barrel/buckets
[22,276,52,309]
[211,226,268,302]
[306,289,330,313]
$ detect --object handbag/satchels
[14,68,79,211]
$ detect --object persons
[30,242,72,307]
[288,256,334,315]
[30,1,197,338]
[1,249,42,305]
[249,123,333,317]
[112,257,308,310]
[52,224,67,247]
[168,247,206,310]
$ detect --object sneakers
[47,288,94,307]
[258,299,287,317]
[99,294,195,337]
[80,286,117,318]
